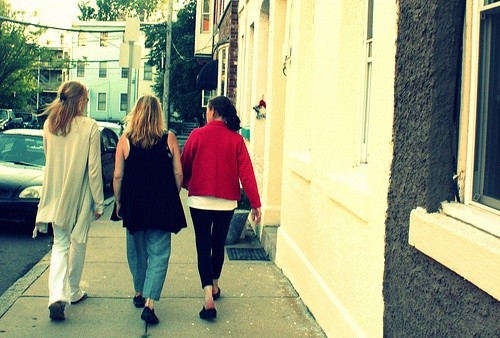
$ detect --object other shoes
[134,295,145,308]
[71,291,87,304]
[141,307,159,324]
[49,301,65,320]
[200,305,217,319]
[212,287,220,301]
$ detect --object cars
[181,121,201,136]
[96,120,126,138]
[98,125,118,195]
[0,128,53,233]
[0,108,38,132]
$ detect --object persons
[32,81,104,320]
[180,95,262,319]
[110,95,187,334]
